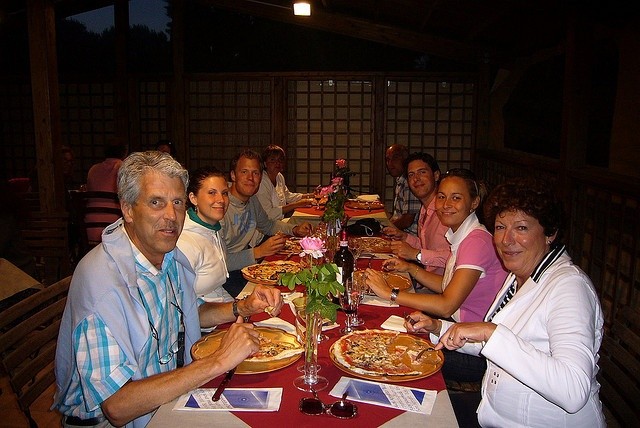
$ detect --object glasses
[299,389,357,418]
[149,301,185,363]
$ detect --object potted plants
[293,296,332,392]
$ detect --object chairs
[598,311,640,427]
[1,276,107,427]
[72,190,120,258]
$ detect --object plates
[277,237,307,257]
[353,270,416,295]
[329,330,445,383]
[352,235,394,254]
[241,260,303,285]
[346,202,385,210]
[191,327,304,374]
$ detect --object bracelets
[232,297,250,323]
[391,285,400,302]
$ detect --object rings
[446,336,453,341]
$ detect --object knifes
[403,311,441,337]
[211,366,237,401]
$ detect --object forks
[367,255,374,294]
[416,338,470,359]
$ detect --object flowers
[279,236,347,325]
[314,157,355,216]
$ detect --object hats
[346,216,381,236]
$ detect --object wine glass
[314,188,322,210]
[339,282,358,335]
[346,276,366,328]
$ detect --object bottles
[333,229,354,312]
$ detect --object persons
[259,142,322,220]
[365,169,511,323]
[223,149,314,272]
[85,146,130,243]
[374,143,422,236]
[155,143,177,158]
[53,149,283,428]
[181,167,235,334]
[382,154,467,267]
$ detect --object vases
[325,215,340,267]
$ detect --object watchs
[415,248,423,266]
[402,181,610,427]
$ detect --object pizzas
[350,237,392,254]
[240,261,302,282]
[351,269,386,291]
[352,200,384,211]
[276,235,314,255]
[333,328,442,378]
[244,335,305,362]
